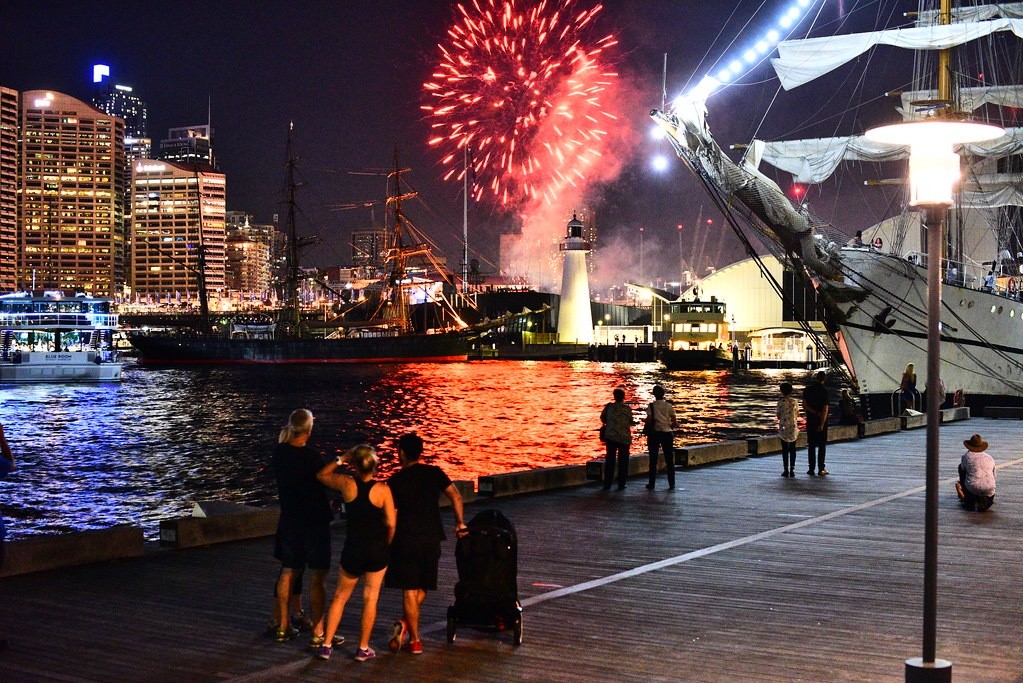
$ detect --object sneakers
[401,639,422,654]
[315,644,332,659]
[277,624,299,641]
[389,619,407,653]
[355,647,376,661]
[310,634,345,647]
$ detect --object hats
[963,434,988,452]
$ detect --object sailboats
[124,119,558,368]
[647,0,1023,421]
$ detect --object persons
[956,434,997,510]
[802,372,828,475]
[949,264,957,286]
[777,383,799,477]
[0,424,15,564]
[908,255,920,265]
[1005,278,1017,300]
[839,390,856,416]
[872,238,883,251]
[853,230,869,248]
[317,444,396,661]
[901,364,917,409]
[600,389,633,490]
[268,409,345,647]
[386,435,467,654]
[646,386,677,489]
[954,390,965,407]
[924,380,946,407]
[984,271,994,293]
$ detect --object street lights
[858,115,1006,683]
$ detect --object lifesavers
[1008,277,1016,293]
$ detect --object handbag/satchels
[642,403,656,434]
[599,426,606,442]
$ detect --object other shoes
[293,610,313,629]
[646,484,655,489]
[790,471,795,477]
[619,485,626,490]
[781,470,789,477]
[670,484,675,489]
[819,468,828,475]
[267,617,281,631]
[807,469,814,475]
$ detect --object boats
[658,284,741,370]
[0,293,126,384]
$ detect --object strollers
[447,513,524,650]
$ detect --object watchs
[336,456,343,465]
